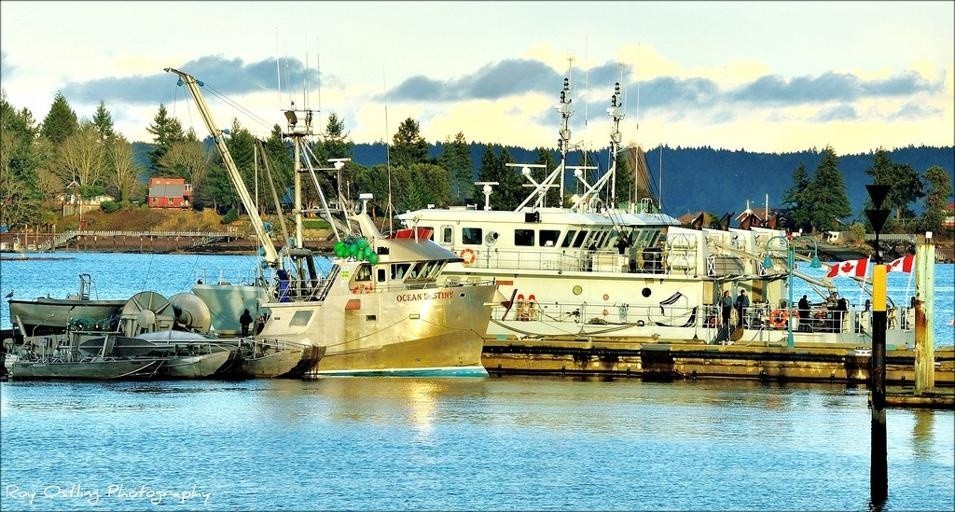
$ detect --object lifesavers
[460,248,476,265]
[769,310,787,327]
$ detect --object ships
[391,54,916,347]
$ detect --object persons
[718,290,732,323]
[835,298,847,333]
[735,289,751,327]
[797,295,809,332]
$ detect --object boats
[163,70,496,377]
[4,272,325,383]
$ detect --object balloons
[333,237,380,268]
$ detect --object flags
[824,258,867,279]
[887,255,915,273]
